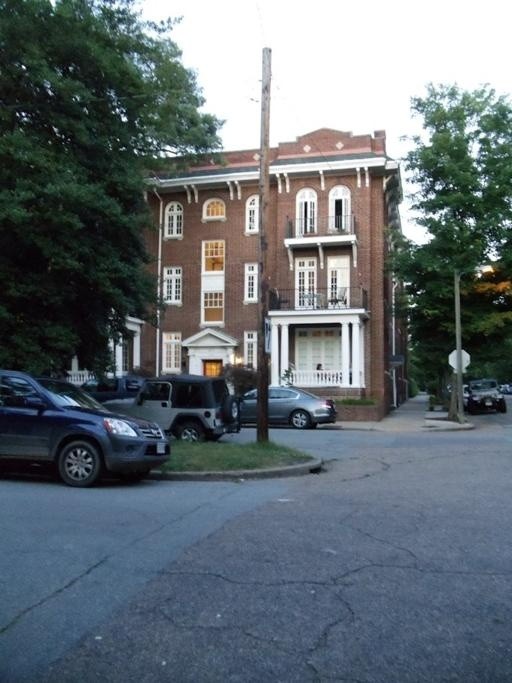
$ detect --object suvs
[104,376,240,443]
[80,376,144,402]
[0,369,173,486]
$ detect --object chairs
[273,288,290,310]
[328,286,348,309]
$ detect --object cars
[239,385,335,430]
[446,378,512,413]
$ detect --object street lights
[453,265,496,424]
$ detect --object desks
[298,293,327,310]
